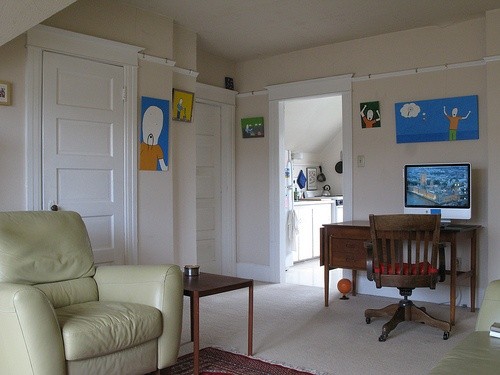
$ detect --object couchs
[0,211,183,375]
[426,280,500,375]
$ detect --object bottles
[294,188,299,199]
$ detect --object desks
[319,220,482,328]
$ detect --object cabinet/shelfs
[290,205,331,265]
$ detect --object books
[489,322,500,338]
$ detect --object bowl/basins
[304,191,315,198]
[184,265,200,276]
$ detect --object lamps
[291,152,304,161]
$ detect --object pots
[317,165,326,182]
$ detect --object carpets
[145,347,318,374]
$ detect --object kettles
[322,185,331,195]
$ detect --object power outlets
[357,155,368,168]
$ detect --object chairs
[365,214,452,341]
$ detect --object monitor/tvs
[402,162,472,223]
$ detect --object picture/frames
[308,168,317,190]
[171,88,195,124]
[0,80,14,109]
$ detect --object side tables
[183,272,254,372]
